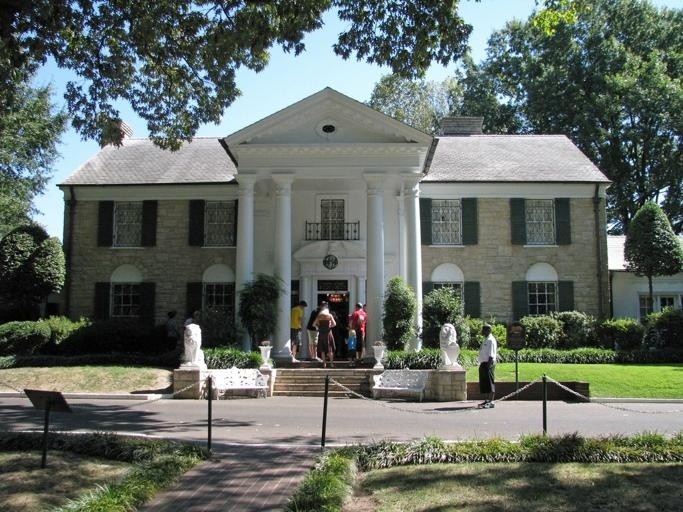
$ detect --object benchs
[207,368,269,400]
[372,369,425,402]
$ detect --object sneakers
[478,403,495,410]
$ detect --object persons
[290,300,307,363]
[477,325,497,408]
[306,299,367,368]
